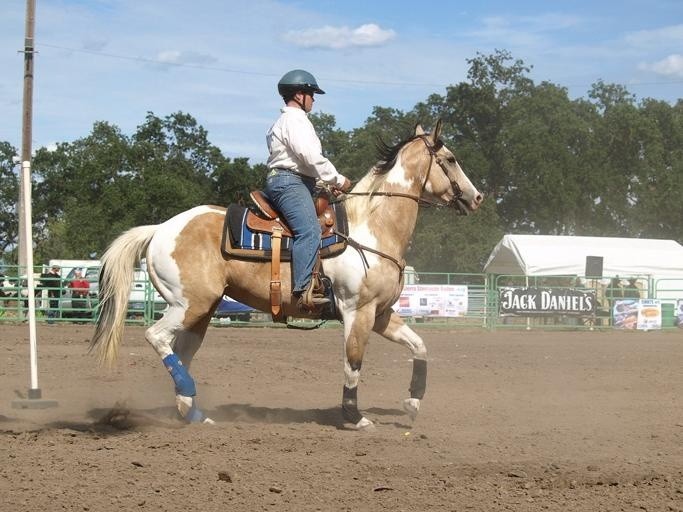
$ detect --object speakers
[586,256,603,279]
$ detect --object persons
[262,65,353,311]
[622,275,641,300]
[66,268,90,325]
[45,264,66,327]
[604,276,623,326]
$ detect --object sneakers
[297,292,331,307]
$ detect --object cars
[35,265,101,315]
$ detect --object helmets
[278,69,324,94]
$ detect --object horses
[0,274,66,322]
[496,275,646,330]
[85,117,484,430]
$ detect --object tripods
[576,279,605,332]
